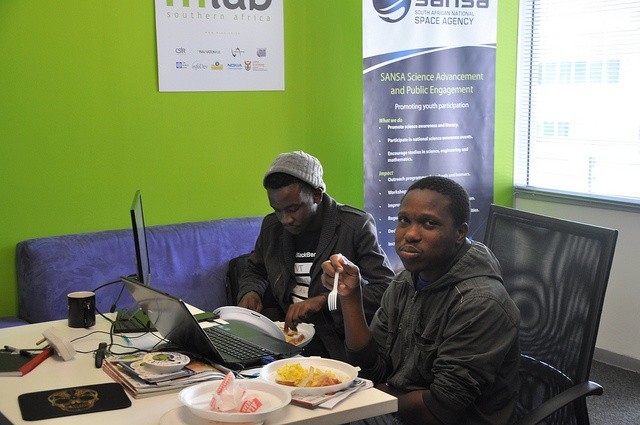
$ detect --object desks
[0,299,400,424]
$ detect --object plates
[178,378,292,423]
[261,356,361,395]
[273,322,315,348]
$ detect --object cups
[67,291,96,328]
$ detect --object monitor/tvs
[115,189,155,333]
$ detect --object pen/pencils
[4,346,32,356]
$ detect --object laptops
[119,276,305,370]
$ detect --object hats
[263,150,327,193]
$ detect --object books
[2,346,54,376]
[103,356,227,398]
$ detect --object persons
[236,150,393,365]
[322,176,521,425]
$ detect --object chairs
[478,204,619,425]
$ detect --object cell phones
[192,312,219,322]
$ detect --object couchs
[0,217,264,327]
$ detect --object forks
[327,253,340,311]
